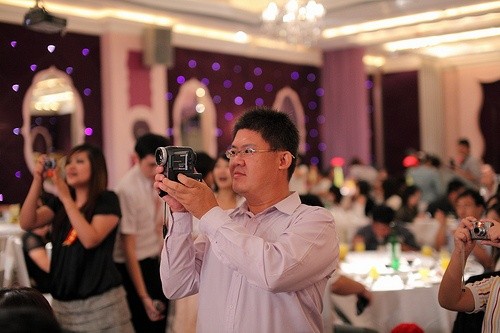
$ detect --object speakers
[143,25,171,65]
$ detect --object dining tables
[332,247,484,333]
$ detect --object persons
[20,145,136,333]
[153,109,340,333]
[405,149,500,272]
[289,155,389,216]
[354,206,422,251]
[299,194,376,333]
[376,186,423,223]
[452,138,482,182]
[438,216,500,333]
[22,225,52,293]
[112,133,171,333]
[166,153,245,333]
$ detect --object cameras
[466,221,494,240]
[155,146,202,183]
[44,158,56,177]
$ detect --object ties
[150,180,169,240]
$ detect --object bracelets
[141,296,151,299]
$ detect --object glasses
[224,147,274,159]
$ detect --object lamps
[25,0,66,35]
